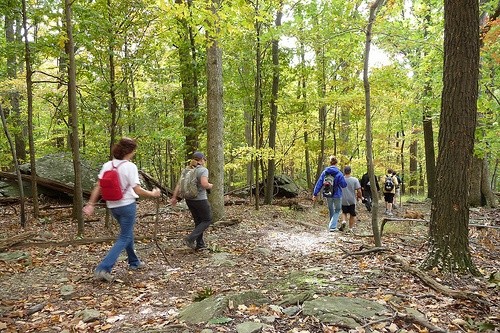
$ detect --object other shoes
[128,260,146,272]
[94,269,115,283]
[366,207,372,212]
[385,210,393,216]
[329,228,337,232]
[196,246,210,253]
[339,222,346,231]
[183,237,198,250]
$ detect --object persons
[168,153,213,250]
[314,158,348,231]
[382,169,402,215]
[339,167,361,233]
[359,172,379,211]
[81,138,160,282]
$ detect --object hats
[192,152,208,161]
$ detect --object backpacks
[385,175,394,191]
[99,160,129,201]
[321,171,338,199]
[395,176,402,186]
[180,165,202,200]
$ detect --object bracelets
[87,202,92,205]
